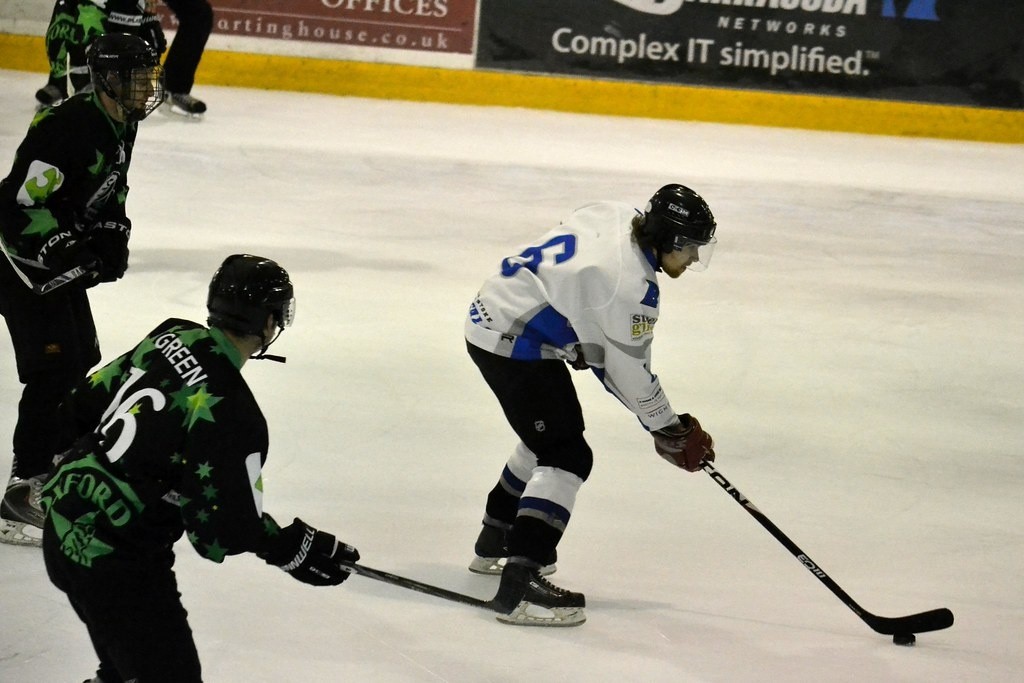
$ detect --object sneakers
[0,475,46,548]
[496,563,586,627]
[156,88,208,124]
[33,83,63,112]
[468,524,557,576]
[52,448,74,468]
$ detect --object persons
[31,0,213,122]
[465,183,715,627]
[50,254,361,683]
[0,32,164,547]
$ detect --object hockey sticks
[351,562,530,614]
[701,461,955,634]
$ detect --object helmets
[645,184,717,251]
[85,33,168,121]
[206,254,293,336]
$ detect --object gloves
[256,518,360,586]
[650,413,716,472]
[37,227,100,298]
[82,214,131,283]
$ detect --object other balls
[893,633,915,646]
